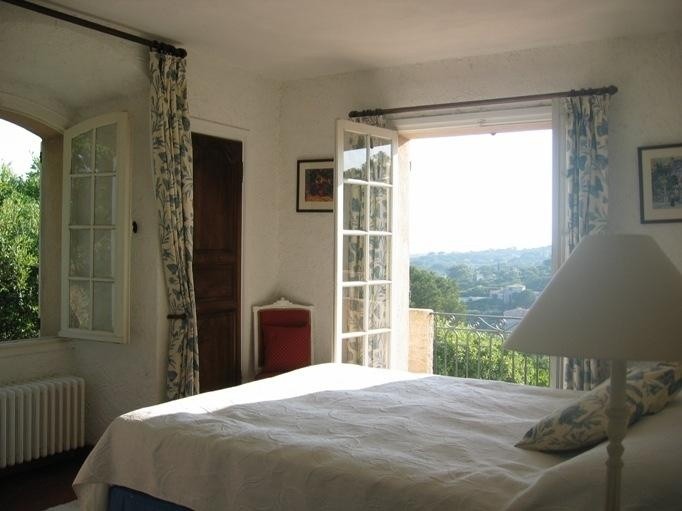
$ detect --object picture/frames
[640,142,682,222]
[295,159,335,212]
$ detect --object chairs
[252,297,314,380]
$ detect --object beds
[73,362,682,511]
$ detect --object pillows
[264,327,311,376]
[514,363,678,451]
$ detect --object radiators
[0,374,84,472]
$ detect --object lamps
[503,230,682,511]
